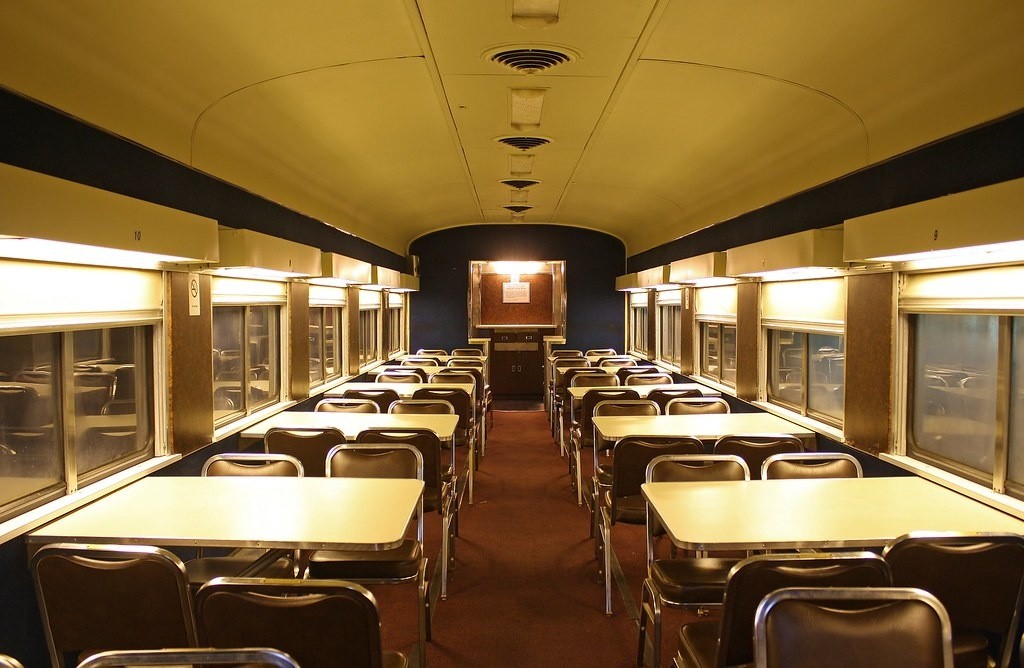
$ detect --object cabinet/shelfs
[474,324,558,400]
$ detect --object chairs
[760,452,865,479]
[598,436,703,616]
[193,576,410,668]
[0,323,334,480]
[751,587,955,668]
[666,552,894,668]
[881,532,1023,667]
[29,543,201,668]
[181,454,303,594]
[263,427,347,478]
[303,443,433,668]
[637,454,750,668]
[546,349,731,509]
[312,348,491,413]
[703,326,997,475]
[714,434,805,480]
[357,426,456,599]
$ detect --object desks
[590,413,814,440]
[640,476,1024,552]
[28,475,425,575]
[242,411,460,440]
